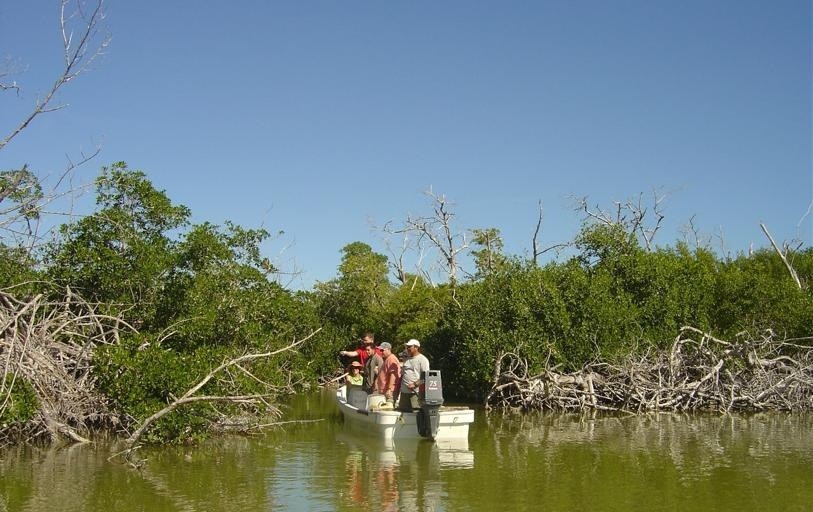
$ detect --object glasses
[380,349,384,352]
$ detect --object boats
[332,407,479,476]
[333,367,475,438]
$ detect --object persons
[338,332,431,412]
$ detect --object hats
[374,342,391,350]
[346,361,362,368]
[403,338,421,347]
